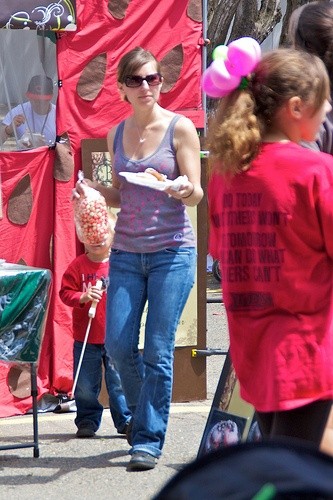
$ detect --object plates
[119,172,174,191]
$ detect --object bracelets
[4,126,11,135]
[182,187,194,198]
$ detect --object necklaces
[31,106,51,133]
[132,120,148,142]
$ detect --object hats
[26,76,53,100]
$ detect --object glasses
[124,73,162,88]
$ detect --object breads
[136,167,165,182]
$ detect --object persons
[71,48,205,471]
[201,36,333,451]
[0,74,57,147]
[288,0,333,155]
[59,210,132,437]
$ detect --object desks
[0,262,52,457]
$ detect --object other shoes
[118,423,128,434]
[126,418,133,446]
[76,427,94,437]
[128,449,156,469]
